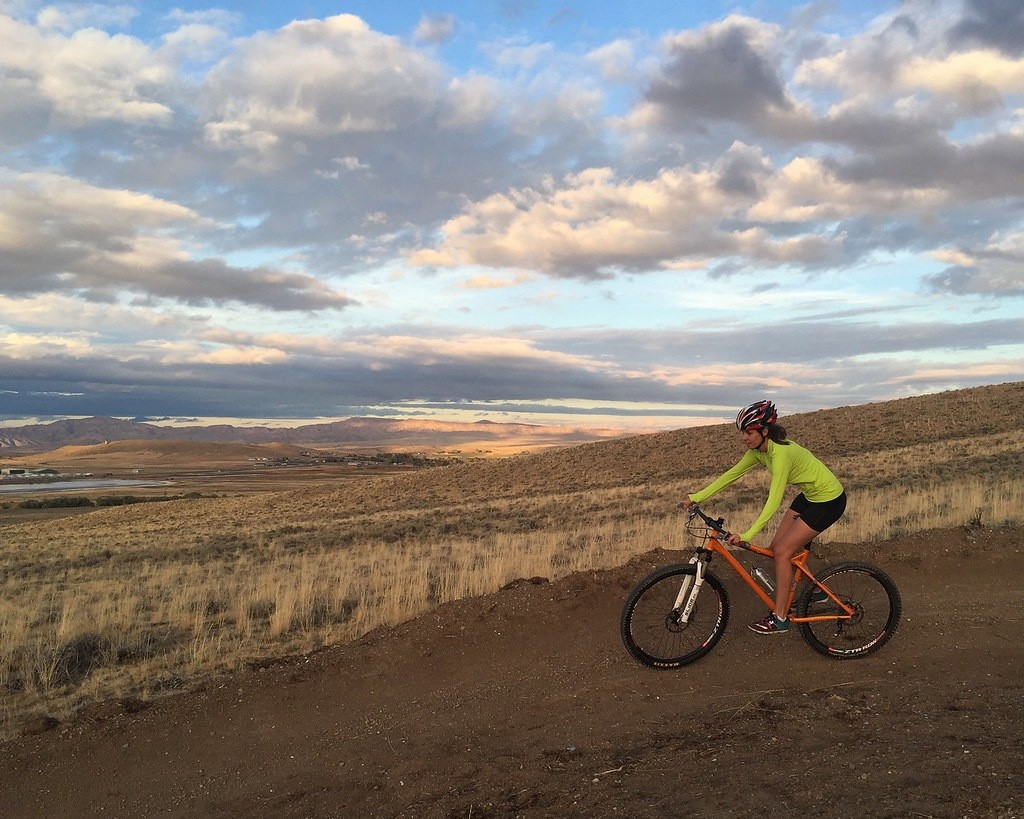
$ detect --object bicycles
[619,501,902,671]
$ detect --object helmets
[735,400,778,431]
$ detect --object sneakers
[747,610,790,634]
[790,589,829,610]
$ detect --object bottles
[750,564,776,596]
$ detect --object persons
[682,399,847,635]
